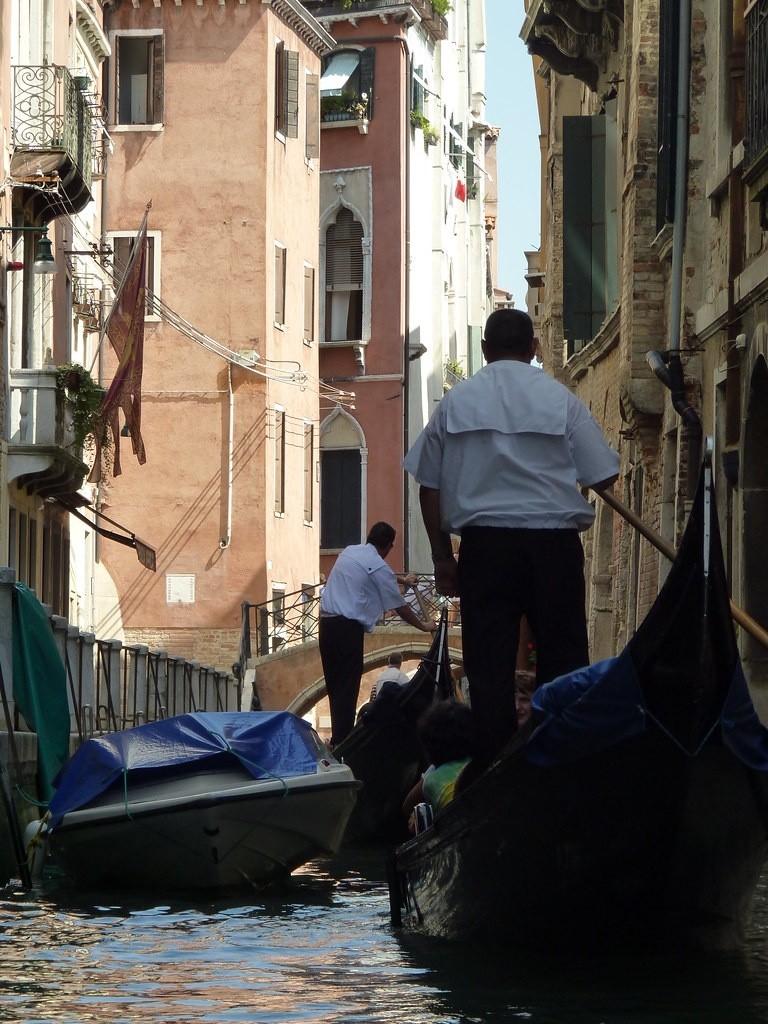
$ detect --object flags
[456,179,466,204]
[85,228,149,482]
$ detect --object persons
[319,522,436,749]
[403,308,624,765]
[402,699,473,814]
[319,574,328,587]
[515,674,538,729]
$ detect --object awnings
[320,51,361,97]
[79,502,159,570]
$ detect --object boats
[387,449,767,952]
[328,607,465,852]
[22,711,364,896]
